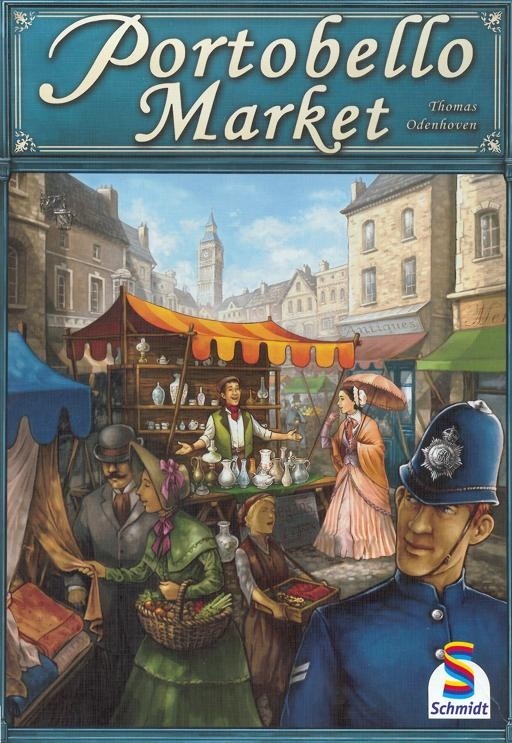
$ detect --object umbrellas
[343,373,408,439]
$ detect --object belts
[230,446,245,453]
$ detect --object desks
[170,474,338,538]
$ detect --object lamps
[38,191,72,232]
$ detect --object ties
[347,418,353,440]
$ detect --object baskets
[134,578,232,653]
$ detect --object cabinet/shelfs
[106,364,284,459]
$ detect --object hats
[398,399,505,508]
[237,491,271,528]
[126,439,192,513]
[215,375,239,394]
[351,385,367,407]
[92,423,144,462]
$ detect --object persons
[280,401,508,728]
[175,375,303,475]
[64,424,264,729]
[312,386,396,560]
[235,493,328,729]
[280,399,306,456]
